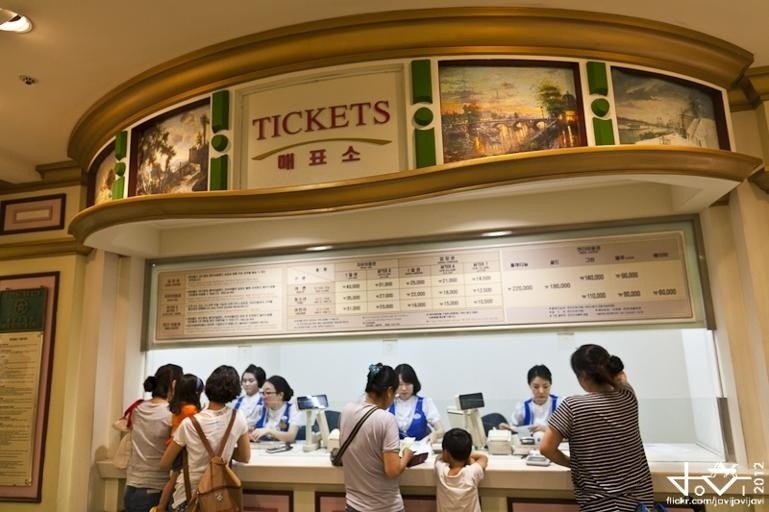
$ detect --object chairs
[481,413,517,437]
[295,411,340,441]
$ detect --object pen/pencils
[521,454,528,459]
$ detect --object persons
[123,364,183,511]
[231,363,266,421]
[433,428,488,512]
[512,365,564,434]
[538,344,655,511]
[339,362,418,512]
[248,374,300,443]
[159,365,251,511]
[385,364,445,447]
[151,374,204,511]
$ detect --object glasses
[260,389,278,398]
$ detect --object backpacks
[182,409,245,512]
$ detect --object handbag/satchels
[329,447,342,466]
[112,416,133,468]
